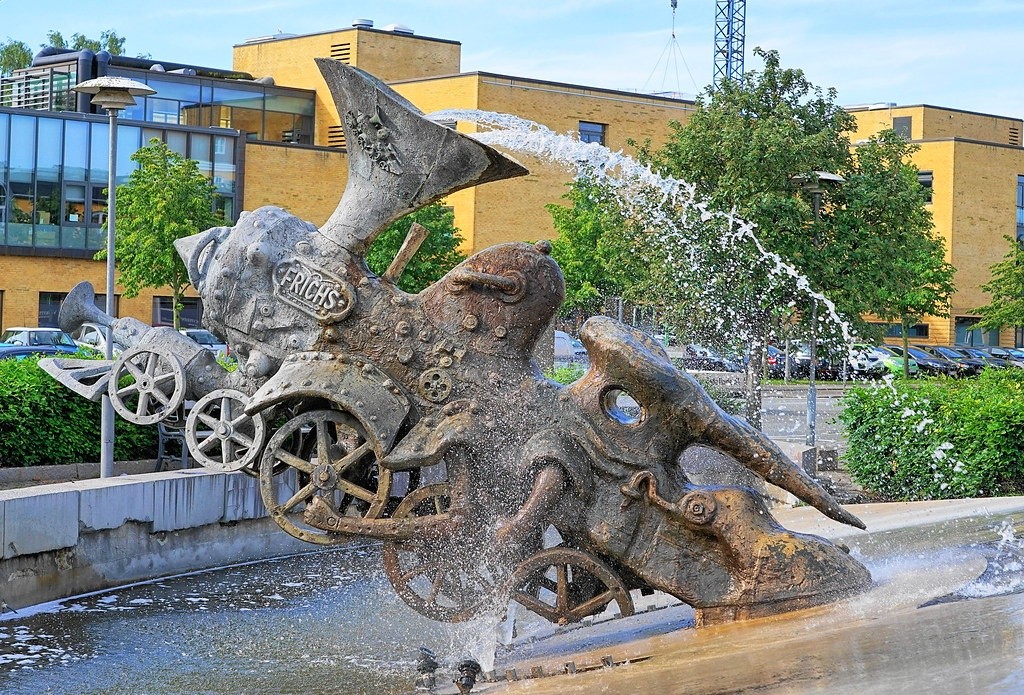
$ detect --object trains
[32,58,872,628]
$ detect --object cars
[666,336,1023,382]
[1,326,79,355]
[178,328,230,360]
[73,323,127,359]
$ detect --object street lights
[71,72,158,480]
